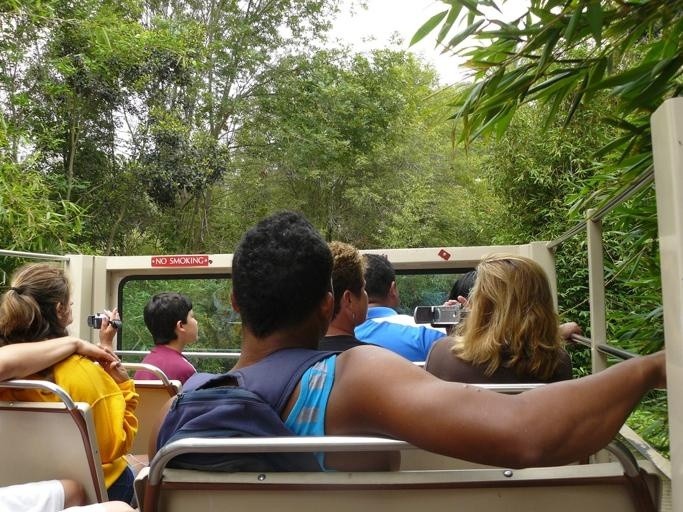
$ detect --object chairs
[0,361,656,512]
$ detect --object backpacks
[154,345,347,473]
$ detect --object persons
[424,253,574,382]
[354,253,447,362]
[0,335,118,382]
[147,210,667,512]
[0,262,149,509]
[133,291,198,385]
[0,479,136,512]
[450,270,583,339]
[317,240,383,352]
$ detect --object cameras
[87,313,122,329]
[414,305,461,326]
